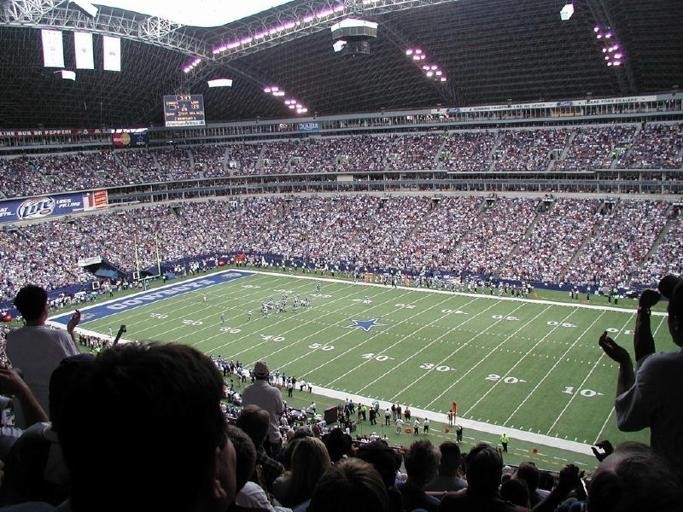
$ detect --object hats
[321,427,353,456]
[658,276,682,307]
[254,362,268,377]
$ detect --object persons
[599,274,680,471]
[234,171,348,276]
[0,131,85,197]
[240,116,407,173]
[586,439,680,510]
[0,210,114,318]
[1,317,233,511]
[347,173,476,287]
[113,181,234,291]
[203,281,325,338]
[476,173,594,301]
[564,99,682,173]
[86,125,239,185]
[386,399,586,511]
[235,359,387,511]
[596,170,683,303]
[407,109,564,172]
